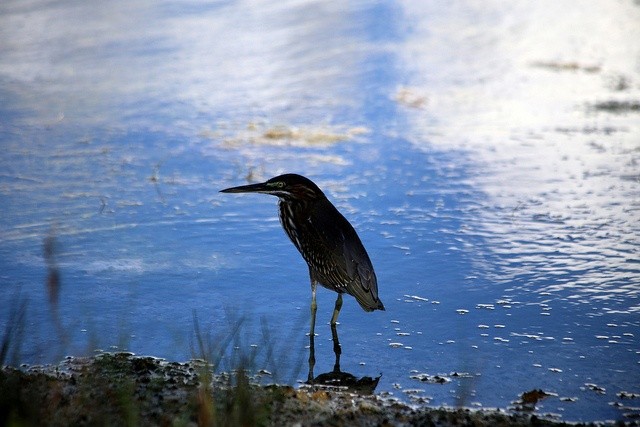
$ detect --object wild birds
[219,173,387,362]
